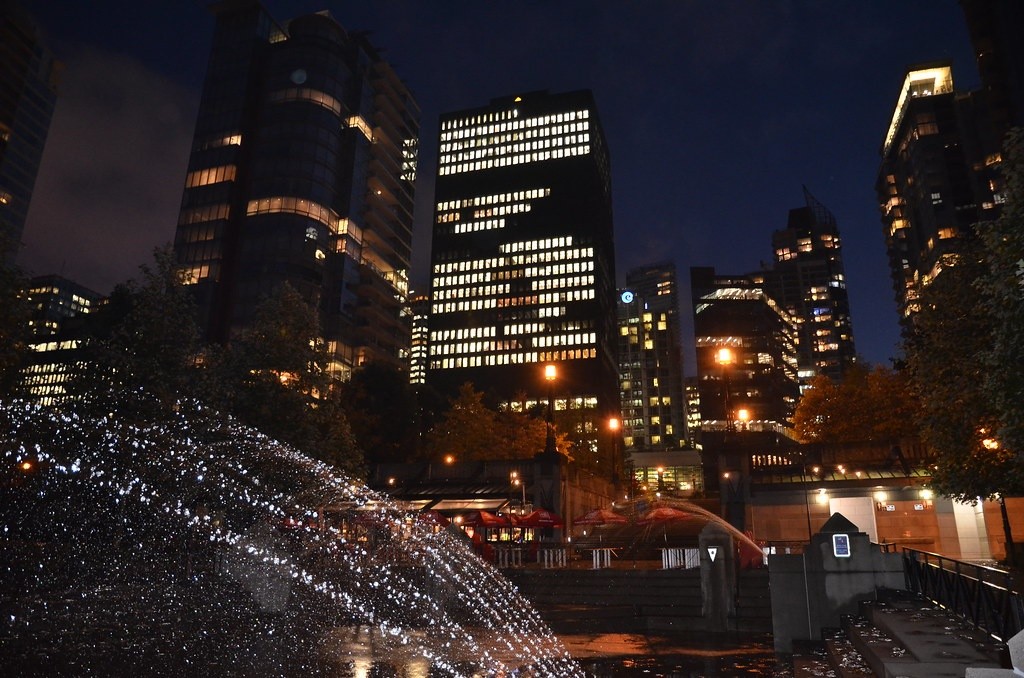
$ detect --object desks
[655,546,704,570]
[533,547,567,570]
[581,547,618,569]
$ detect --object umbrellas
[572,507,692,543]
[355,509,562,545]
